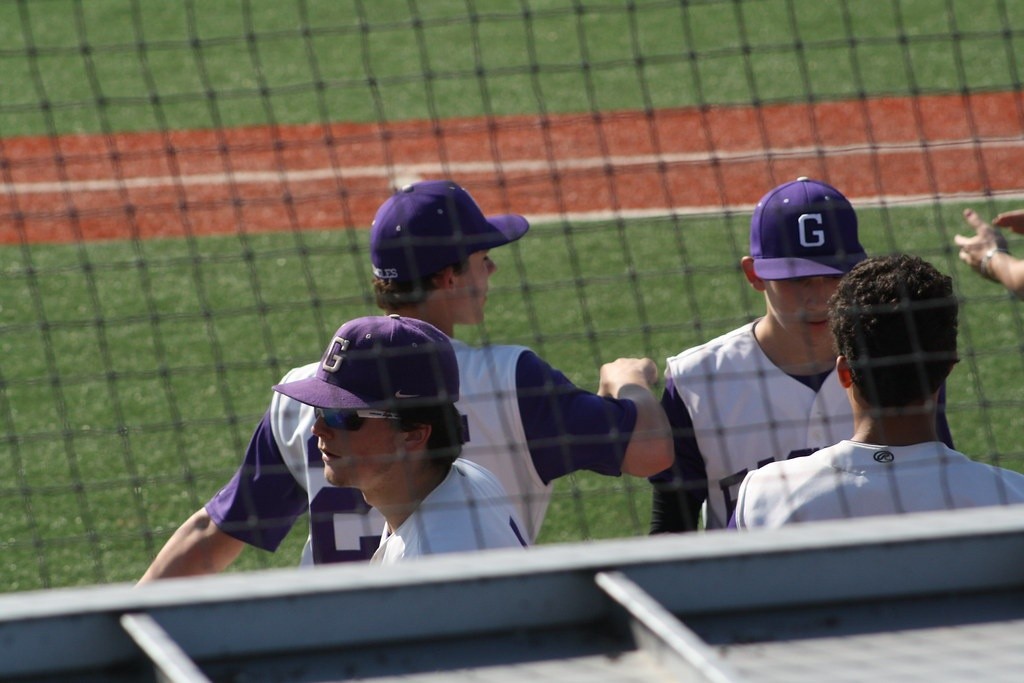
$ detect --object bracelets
[980,248,1009,284]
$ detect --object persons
[648,176,955,542]
[955,208,1024,292]
[271,312,532,564]
[136,180,676,585]
[722,251,1024,526]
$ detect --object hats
[752,175,869,282]
[369,179,528,280]
[271,314,460,412]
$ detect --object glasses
[314,406,404,430]
